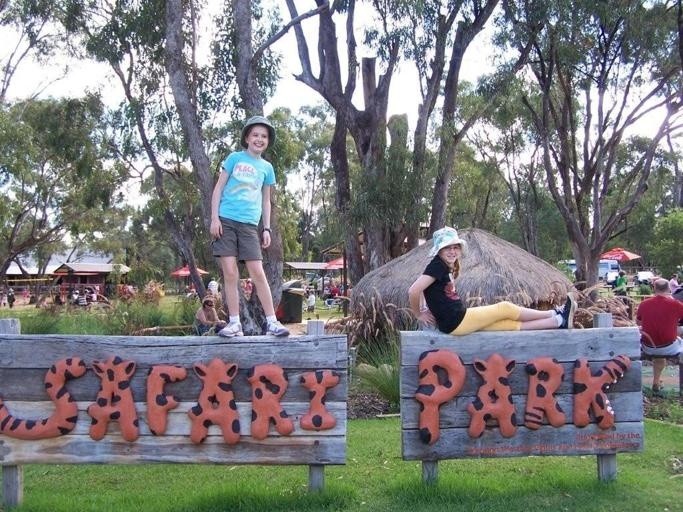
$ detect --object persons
[0,278,166,313]
[187,274,353,336]
[209,116,290,336]
[409,227,577,335]
[575,266,683,397]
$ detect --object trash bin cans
[324,293,333,305]
[282,280,302,323]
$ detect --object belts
[640,340,676,350]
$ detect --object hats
[427,225,469,259]
[240,115,277,149]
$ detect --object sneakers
[553,292,579,329]
[265,319,290,337]
[306,313,320,321]
[652,380,664,392]
[217,321,244,337]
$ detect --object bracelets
[419,303,429,313]
[262,228,272,234]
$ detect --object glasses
[440,246,461,252]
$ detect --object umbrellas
[599,247,642,263]
[169,263,209,293]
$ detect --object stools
[641,351,683,398]
